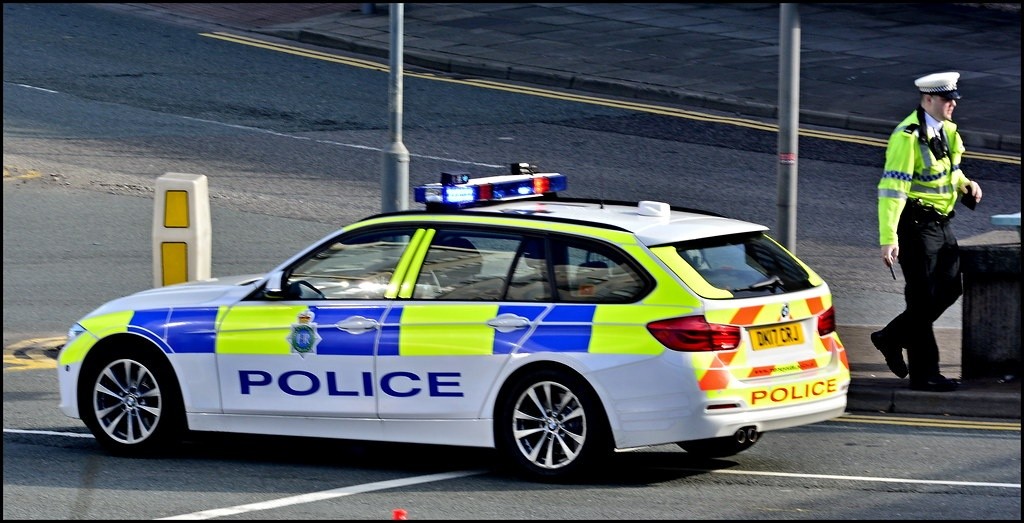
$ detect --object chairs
[512,239,548,283]
[441,238,506,299]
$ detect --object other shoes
[870,326,909,378]
[908,370,957,392]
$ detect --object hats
[914,71,960,100]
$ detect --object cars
[56,161,851,487]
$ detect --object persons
[870,72,983,393]
